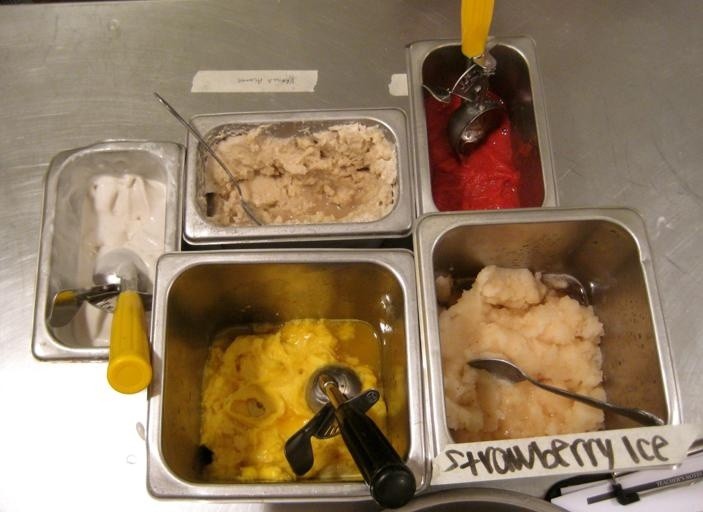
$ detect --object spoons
[151,92,265,223]
[470,357,665,423]
